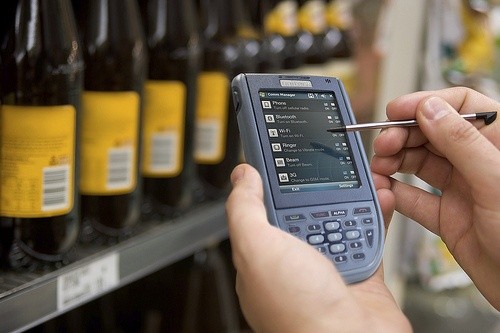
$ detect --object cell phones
[232,72,386,284]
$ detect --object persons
[224,87,500,333]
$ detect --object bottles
[1,1,356,268]
